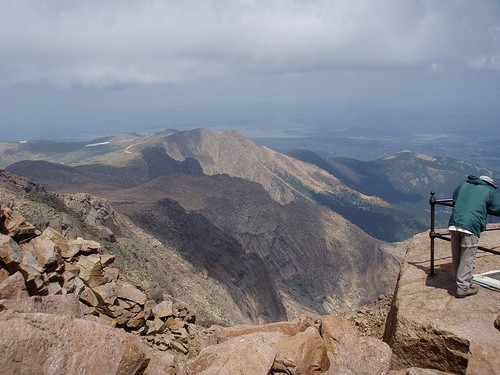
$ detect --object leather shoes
[456,285,478,298]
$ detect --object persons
[446,175,500,298]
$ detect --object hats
[480,176,493,184]
